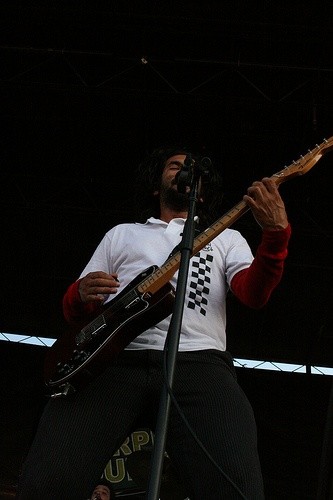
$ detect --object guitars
[43,136,333,401]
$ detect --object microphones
[175,149,196,192]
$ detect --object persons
[88,483,115,500]
[13,147,291,500]
